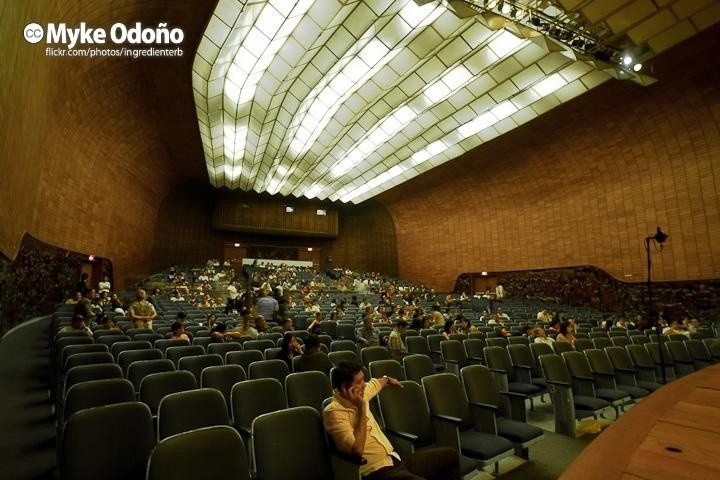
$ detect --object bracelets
[382,375,391,384]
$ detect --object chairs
[44,256,720,480]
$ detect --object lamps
[459,0,649,77]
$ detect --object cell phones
[340,383,362,397]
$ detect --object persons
[53,254,699,377]
[321,361,461,480]
